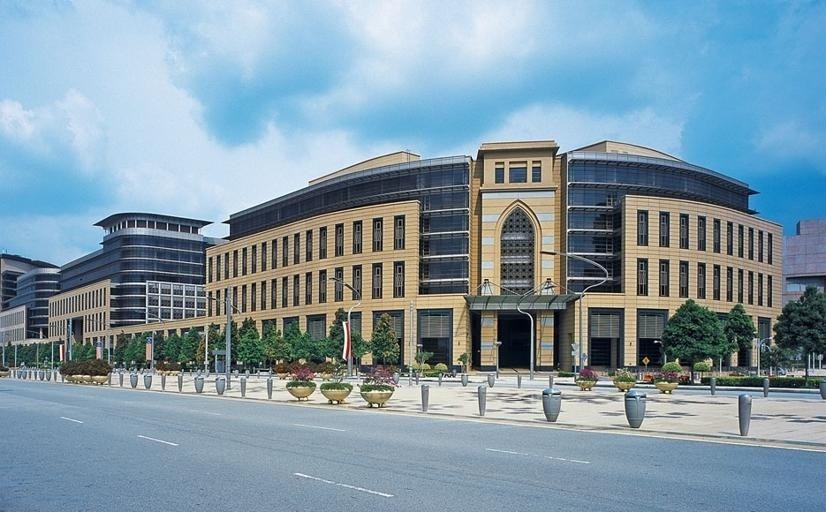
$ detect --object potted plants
[360,384,395,407]
[614,375,636,392]
[0,367,10,378]
[286,382,316,400]
[320,382,353,404]
[575,377,598,391]
[276,361,335,381]
[59,360,112,385]
[155,363,180,376]
[656,377,679,394]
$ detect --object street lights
[494,341,504,381]
[762,343,773,377]
[537,249,611,371]
[327,276,363,377]
[206,296,242,324]
[653,340,667,364]
[0,309,167,369]
[757,337,774,376]
[414,343,425,359]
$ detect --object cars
[763,366,787,375]
